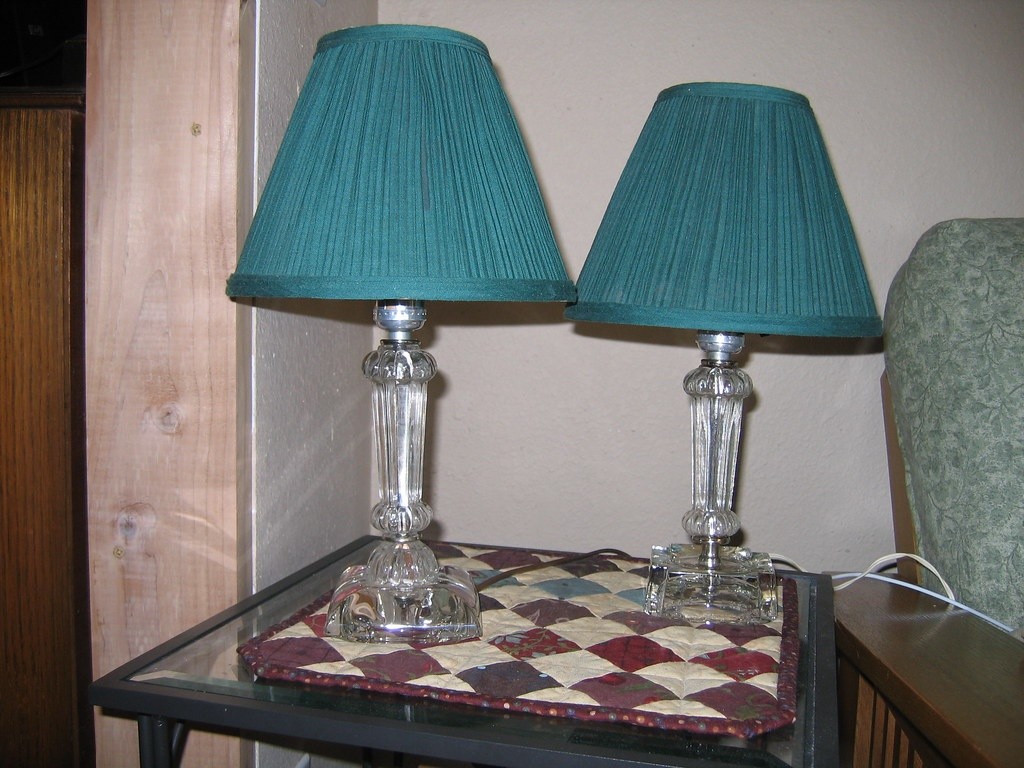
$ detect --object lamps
[226,24,577,641]
[563,81,883,625]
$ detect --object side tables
[91,534,840,768]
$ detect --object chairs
[825,218,1024,768]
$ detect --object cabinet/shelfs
[0,77,95,768]
